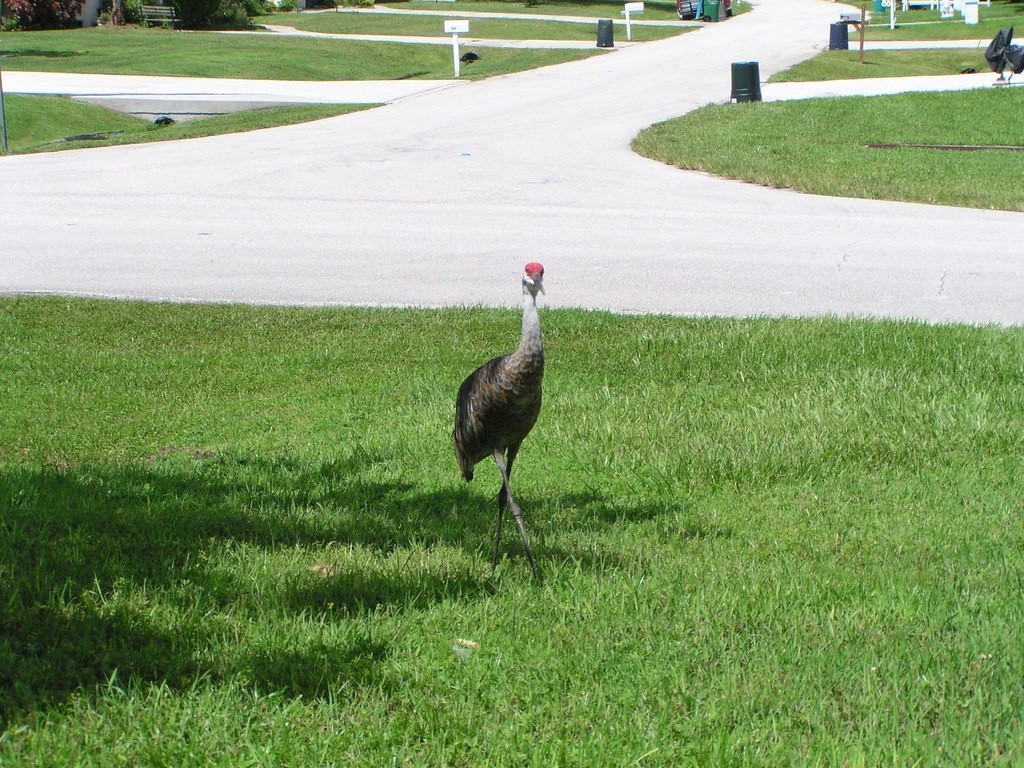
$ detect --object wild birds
[453,261,552,584]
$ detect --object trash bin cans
[703,0,719,23]
